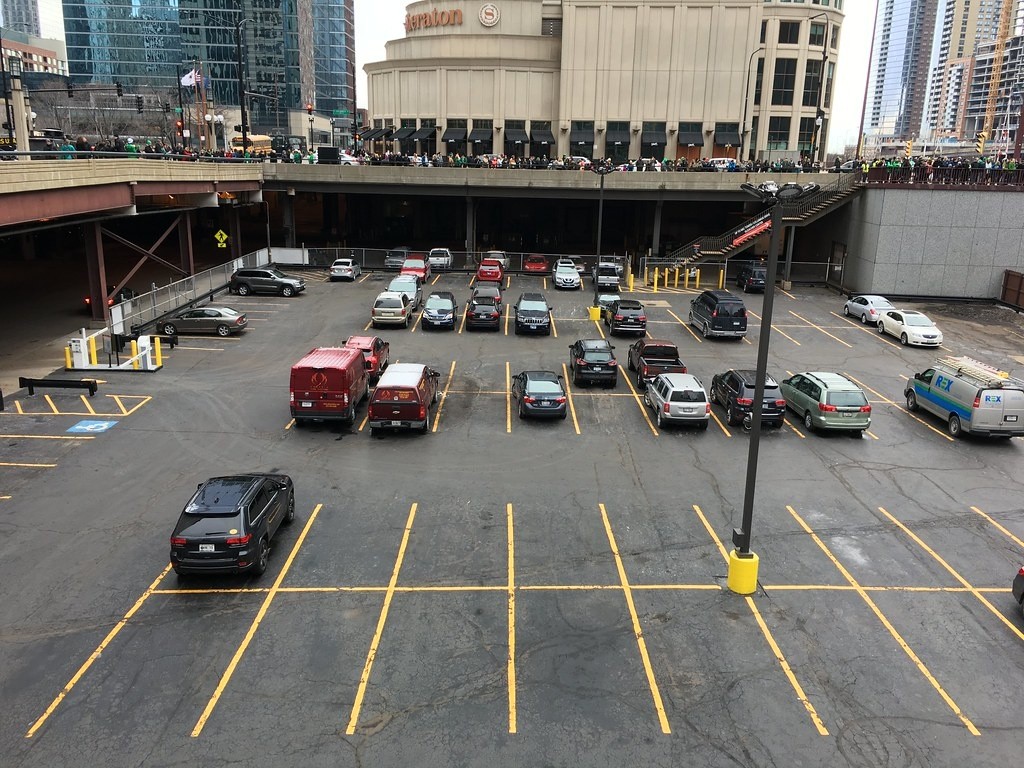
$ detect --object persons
[422,152,429,167]
[727,156,812,173]
[628,160,634,171]
[44,136,314,164]
[346,145,417,166]
[636,156,644,171]
[835,158,840,173]
[852,156,1024,186]
[661,157,722,172]
[432,152,612,170]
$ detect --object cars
[153,247,947,439]
[566,156,592,165]
[312,152,361,167]
[828,159,873,173]
[707,158,736,171]
[615,158,662,172]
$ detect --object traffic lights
[67,83,75,98]
[904,141,912,157]
[176,121,183,136]
[308,104,313,115]
[975,132,988,155]
[350,124,357,135]
[116,82,124,96]
[165,102,171,112]
[355,134,360,140]
[234,124,241,133]
[136,96,144,114]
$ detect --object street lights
[0,22,32,151]
[182,10,256,157]
[345,55,357,158]
[808,12,829,173]
[589,168,615,322]
[727,178,823,595]
[740,47,766,161]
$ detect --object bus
[227,133,308,158]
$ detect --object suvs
[903,355,1024,440]
[169,471,295,576]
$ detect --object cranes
[981,0,1015,141]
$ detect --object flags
[180,69,201,86]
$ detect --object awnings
[505,129,529,144]
[570,130,594,145]
[714,132,740,148]
[442,129,493,142]
[606,130,630,145]
[679,132,704,146]
[642,131,667,146]
[530,130,555,144]
[360,128,435,140]
